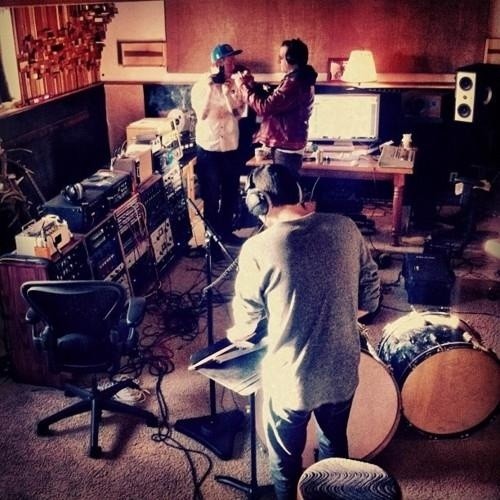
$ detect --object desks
[245,146,417,247]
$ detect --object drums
[376,307,500,435]
[254,322,402,471]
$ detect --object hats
[211,43,243,64]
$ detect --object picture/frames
[326,57,349,83]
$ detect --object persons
[190,41,254,246]
[231,36,318,173]
[229,162,383,499]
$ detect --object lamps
[340,46,379,92]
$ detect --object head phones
[61,182,83,205]
[244,162,305,216]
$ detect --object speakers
[453,62,500,124]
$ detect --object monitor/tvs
[307,89,382,152]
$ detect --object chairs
[15,273,159,461]
